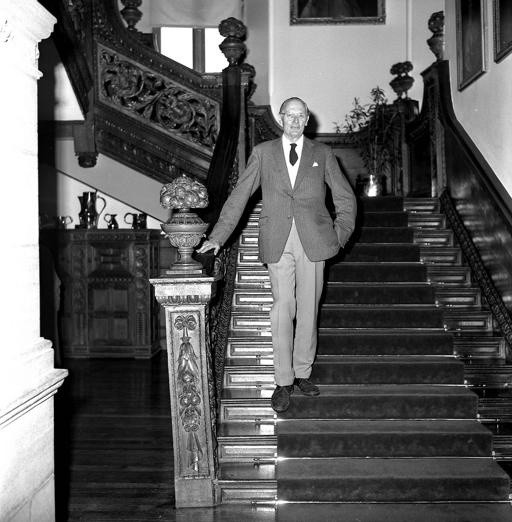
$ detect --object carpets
[289,143,298,165]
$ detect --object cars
[123,213,148,230]
[55,216,73,229]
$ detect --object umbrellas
[294,378,320,397]
[271,385,294,413]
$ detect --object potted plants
[455,0,512,92]
[289,0,386,25]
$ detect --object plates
[51,229,163,360]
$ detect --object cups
[280,112,308,120]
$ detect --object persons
[195,98,358,412]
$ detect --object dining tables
[332,85,403,198]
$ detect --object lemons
[104,213,119,228]
[77,191,106,230]
